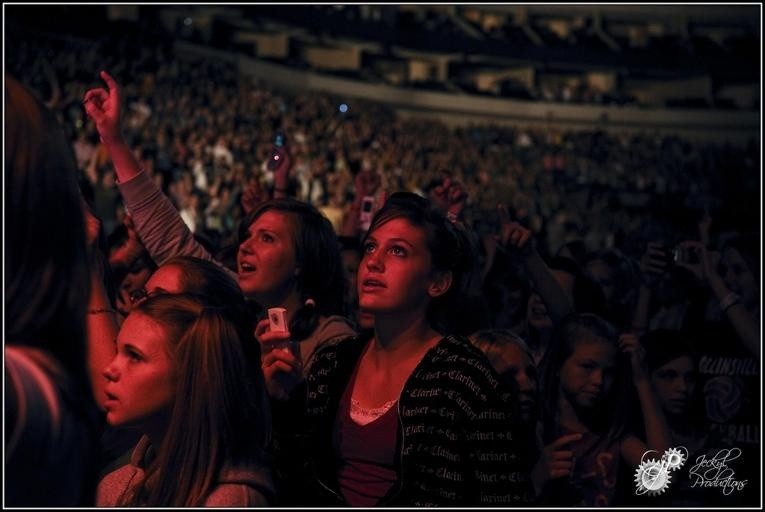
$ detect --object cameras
[664,231,710,269]
[266,309,290,351]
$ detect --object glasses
[130,289,169,306]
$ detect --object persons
[5,5,761,507]
